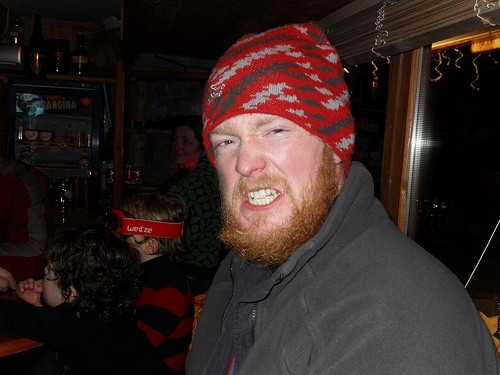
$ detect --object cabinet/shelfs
[7,79,103,216]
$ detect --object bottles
[70,32,89,76]
[10,18,23,45]
[52,182,71,222]
[27,14,46,76]
[51,37,65,74]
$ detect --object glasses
[104,208,127,238]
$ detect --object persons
[0,224,173,375]
[163,114,229,294]
[0,267,17,292]
[184,24,500,375]
[0,94,52,306]
[102,189,194,374]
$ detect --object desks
[0,287,59,374]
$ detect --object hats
[201,23,356,176]
